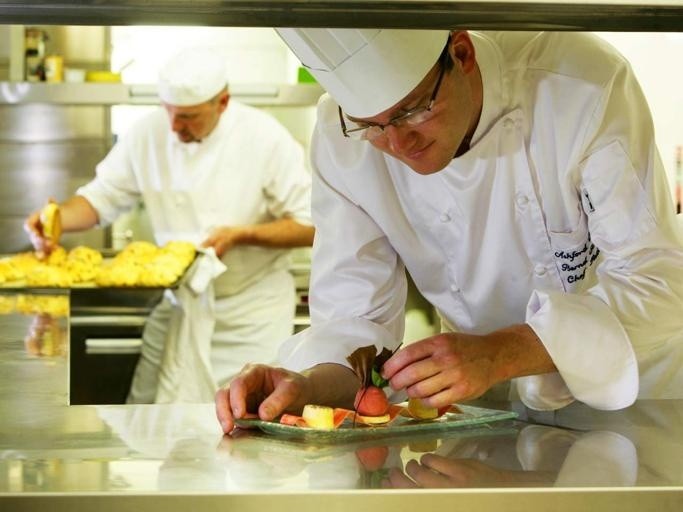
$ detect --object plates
[233,401,520,439]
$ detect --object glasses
[339,63,447,143]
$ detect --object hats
[271,28,450,119]
[159,66,228,107]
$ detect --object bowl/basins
[87,71,122,84]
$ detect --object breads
[0,203,196,288]
[1,294,70,319]
[24,312,58,357]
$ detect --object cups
[64,66,86,82]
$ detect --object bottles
[25,27,63,85]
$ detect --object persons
[23,83,315,405]
[214,30,683,435]
